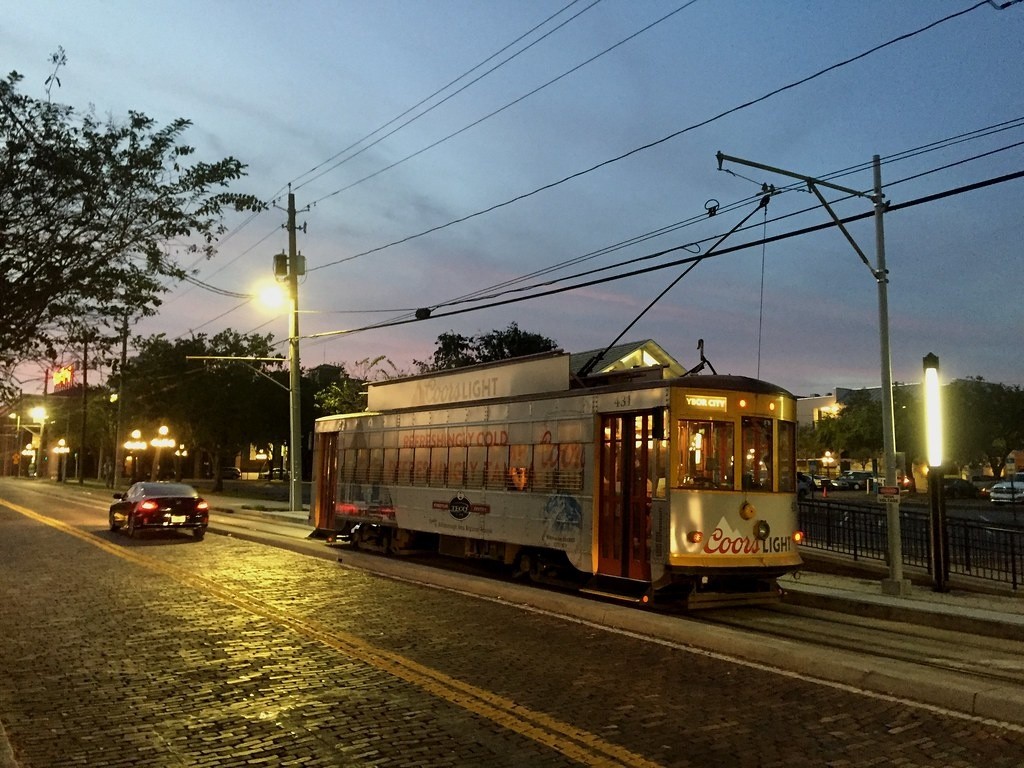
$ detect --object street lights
[821,451,835,477]
[261,289,304,513]
[52,439,70,482]
[123,430,147,486]
[255,449,267,478]
[150,424,176,482]
[22,444,35,476]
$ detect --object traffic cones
[821,486,829,498]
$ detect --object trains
[305,350,805,610]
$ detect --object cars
[941,478,969,498]
[207,466,241,480]
[795,470,909,500]
[258,467,291,480]
[108,482,209,538]
[990,472,1024,506]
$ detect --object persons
[103,456,115,489]
[797,471,810,500]
